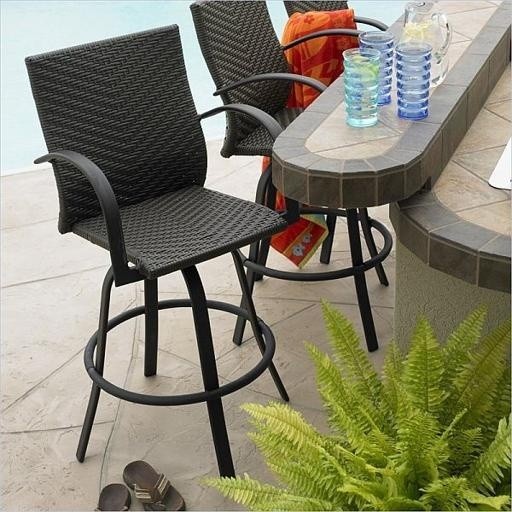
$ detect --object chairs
[262,0,390,33]
[25,25,290,479]
[190,0,392,352]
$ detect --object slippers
[94,483,131,512]
[122,460,186,512]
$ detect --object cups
[360,29,393,102]
[400,2,456,85]
[344,49,383,126]
[394,42,433,122]
